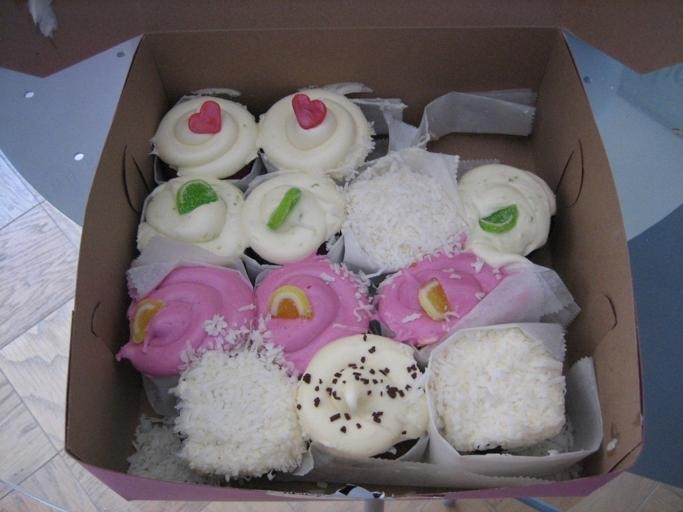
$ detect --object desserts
[115,91,565,481]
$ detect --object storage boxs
[0,0,683,502]
